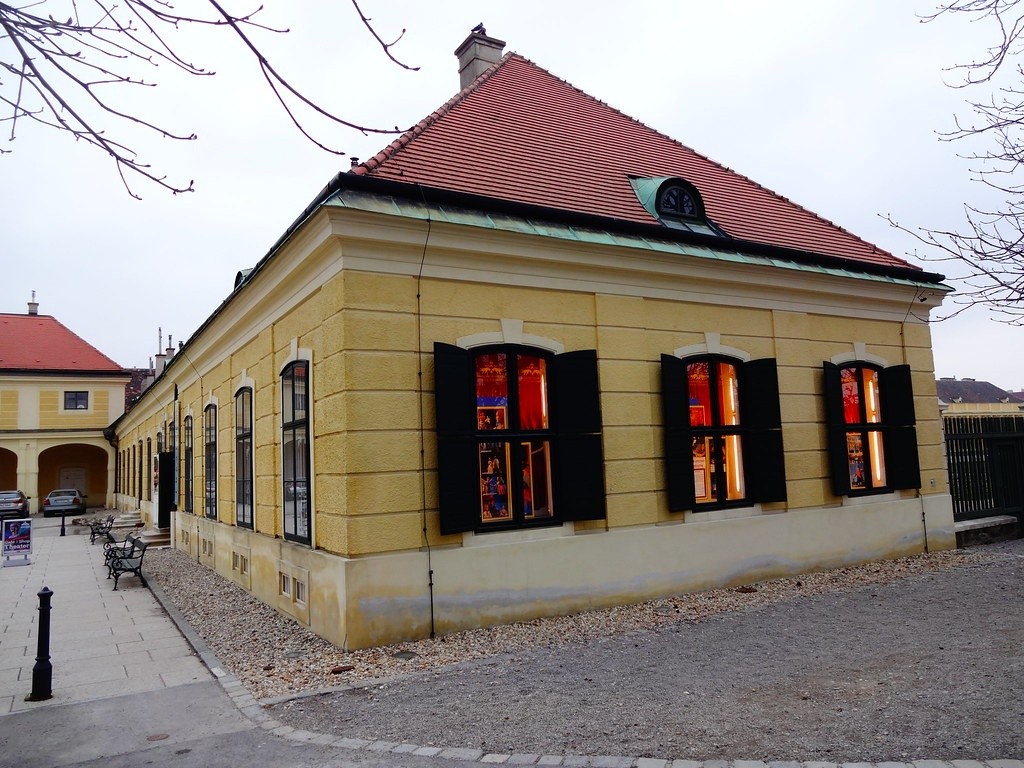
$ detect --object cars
[0,489,31,520]
[42,488,88,518]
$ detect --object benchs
[89,514,115,545]
[103,532,151,591]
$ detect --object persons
[6,524,21,541]
